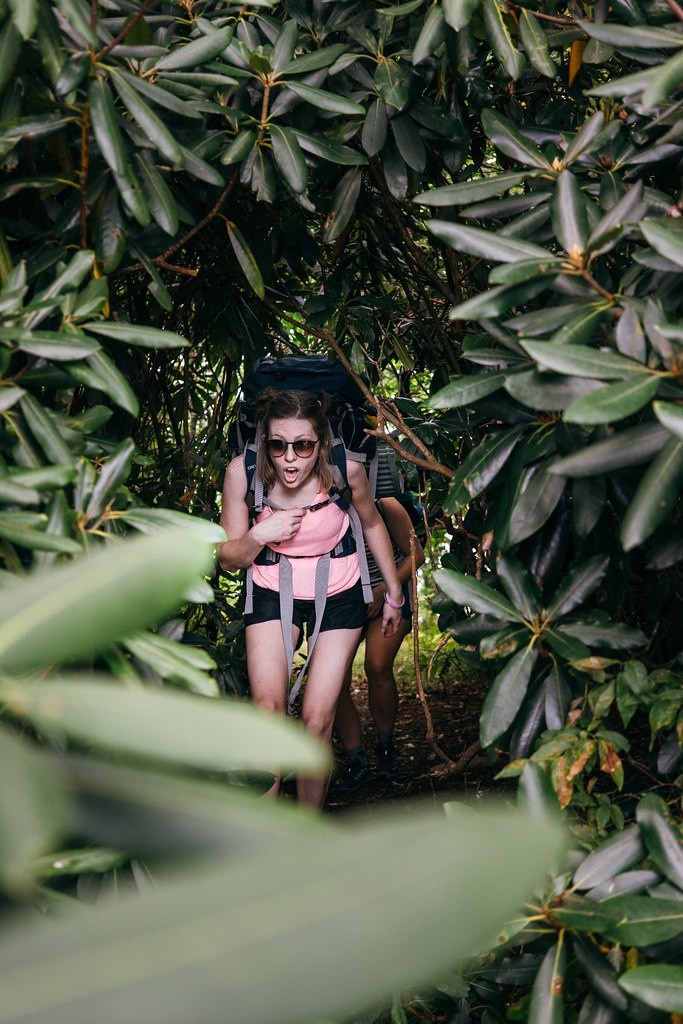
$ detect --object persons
[333,491,426,791]
[219,388,403,809]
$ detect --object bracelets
[386,589,405,608]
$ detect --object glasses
[262,435,319,458]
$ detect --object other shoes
[337,757,372,791]
[375,744,397,778]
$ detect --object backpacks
[230,353,378,513]
